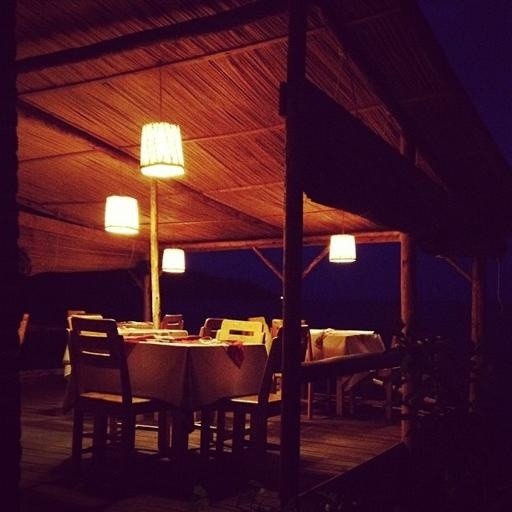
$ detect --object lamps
[103,57,198,274]
[328,198,357,264]
